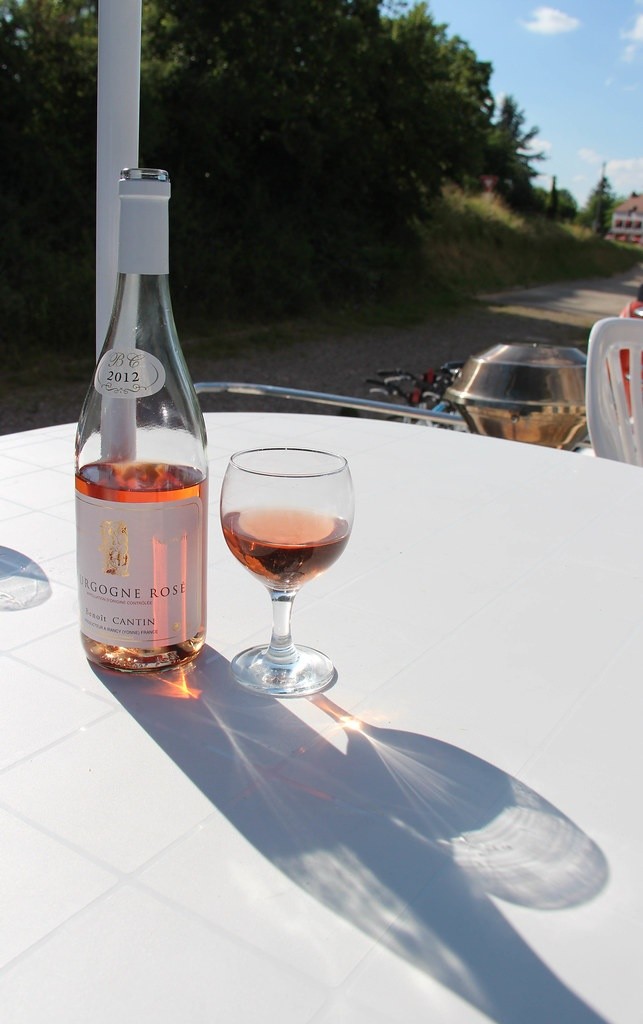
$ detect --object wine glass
[219,448,355,698]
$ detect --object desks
[0,409,642,1023]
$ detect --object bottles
[74,168,211,680]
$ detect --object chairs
[584,315,642,468]
